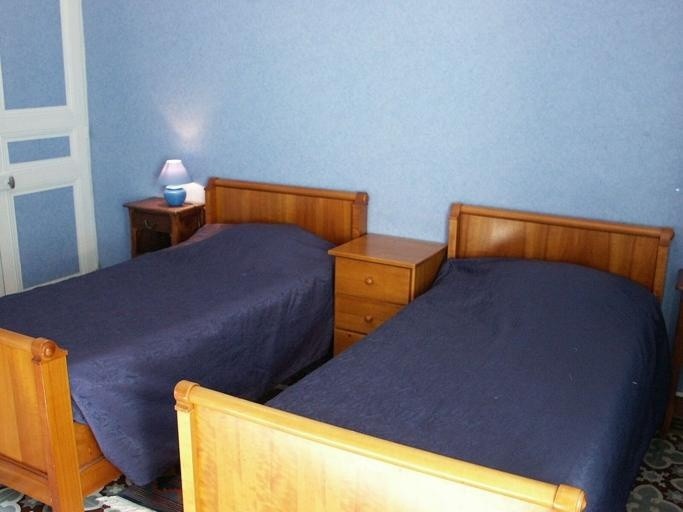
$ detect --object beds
[0,176,369,512]
[174,202,675,512]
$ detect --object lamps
[157,160,192,207]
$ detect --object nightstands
[326,234,448,356]
[662,269,683,436]
[122,197,205,259]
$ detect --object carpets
[96,476,183,512]
